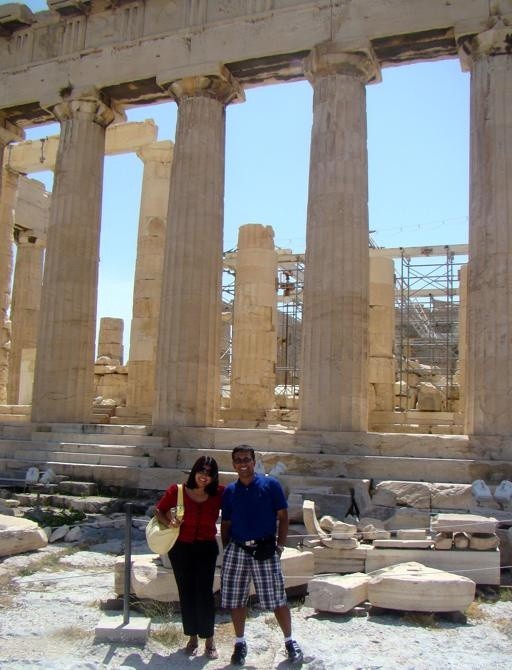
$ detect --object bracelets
[276,544,285,552]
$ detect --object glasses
[234,457,251,464]
[198,468,212,477]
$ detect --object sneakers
[285,640,303,663]
[231,642,247,665]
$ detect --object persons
[153,455,229,660]
[219,444,304,666]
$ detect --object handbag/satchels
[146,484,185,554]
[240,538,276,560]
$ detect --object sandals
[204,647,218,659]
[187,640,197,655]
